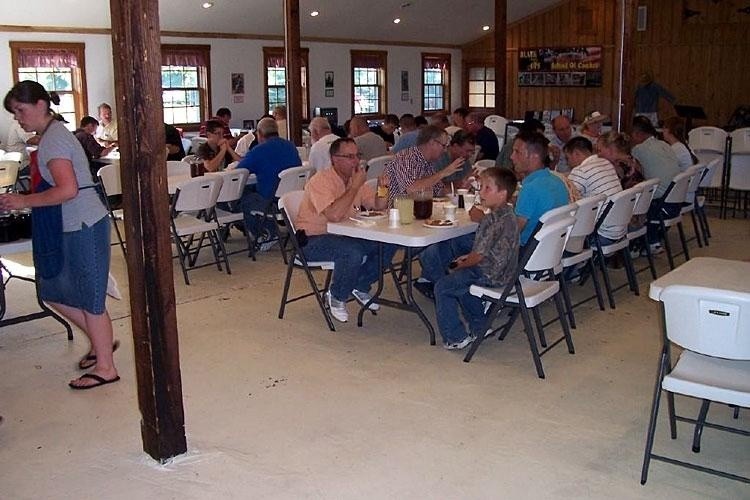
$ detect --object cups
[387,209,403,230]
[393,191,414,224]
[435,188,480,219]
[412,189,434,219]
[189,158,205,177]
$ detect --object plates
[422,219,458,228]
[358,211,386,219]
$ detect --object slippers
[67,342,120,387]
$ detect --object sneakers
[349,289,381,314]
[322,289,350,324]
[442,327,492,351]
[411,278,438,300]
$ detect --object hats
[581,111,609,125]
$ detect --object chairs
[638,280,750,500]
[2,106,748,378]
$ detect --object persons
[190,122,246,243]
[5,116,41,176]
[199,108,233,139]
[163,123,186,161]
[94,103,118,148]
[273,106,288,140]
[0,80,120,389]
[234,114,277,192]
[74,116,121,209]
[236,118,302,253]
[295,109,702,349]
[633,73,676,128]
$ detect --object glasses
[332,152,364,160]
